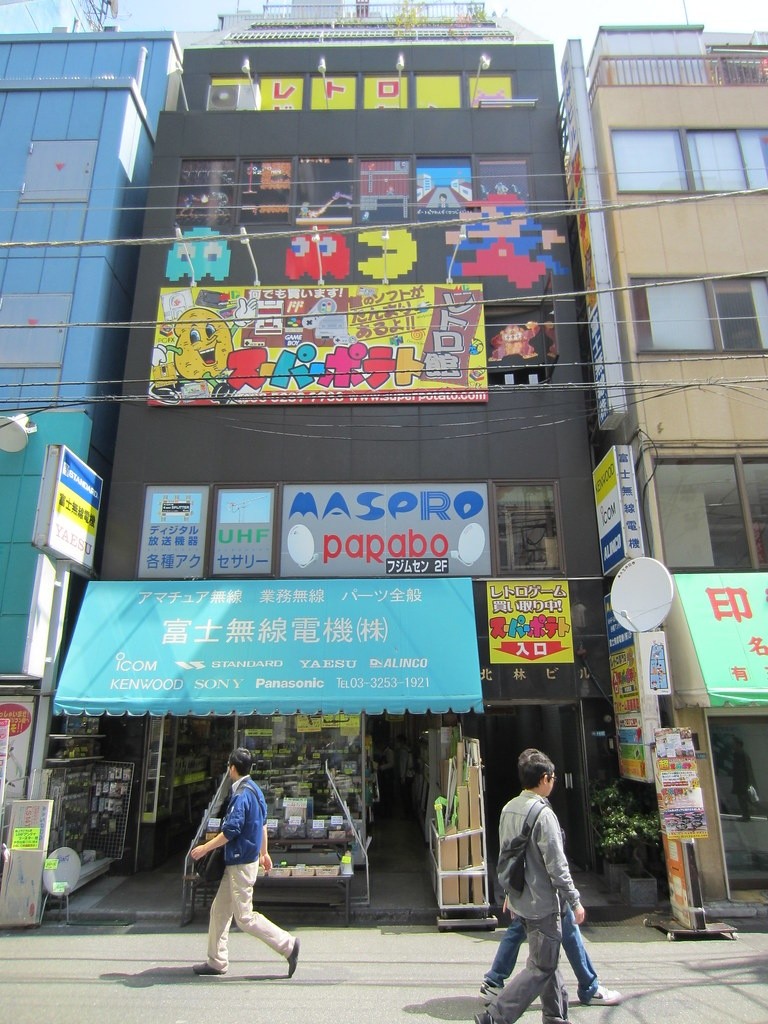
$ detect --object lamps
[396,55,404,109]
[381,229,389,284]
[471,55,489,107]
[242,58,259,111]
[445,225,466,284]
[239,227,261,286]
[318,57,329,109]
[0,411,37,453]
[310,226,324,285]
[175,223,196,287]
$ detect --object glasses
[541,770,559,782]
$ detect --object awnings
[56,577,486,718]
[656,568,768,714]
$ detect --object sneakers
[577,985,622,1005]
[474,1010,497,1024]
[477,981,504,1001]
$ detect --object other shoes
[287,937,301,978]
[191,962,229,975]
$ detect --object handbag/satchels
[194,846,225,882]
[496,838,528,896]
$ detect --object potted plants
[585,771,664,908]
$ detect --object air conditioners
[207,83,261,111]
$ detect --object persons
[190,748,299,979]
[729,736,759,822]
[373,737,416,817]
[475,753,586,1024]
[481,748,623,1008]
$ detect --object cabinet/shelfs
[116,715,377,926]
